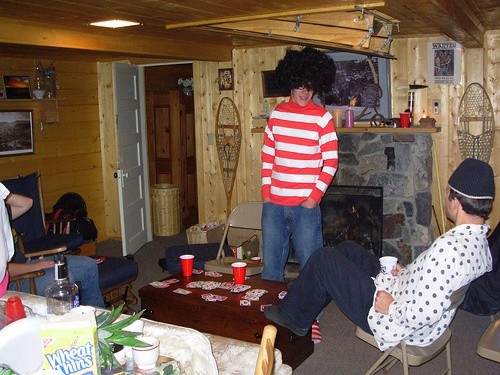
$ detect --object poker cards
[148,269,287,312]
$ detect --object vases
[183,87,193,96]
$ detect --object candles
[345,107,354,128]
[332,109,342,128]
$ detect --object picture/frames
[218,68,234,90]
[0,109,35,157]
[311,51,392,122]
[1,75,33,100]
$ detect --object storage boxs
[42,305,101,375]
[78,240,96,257]
[186,224,225,246]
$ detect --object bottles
[44,255,79,319]
[332,109,343,128]
[345,110,354,128]
[33,64,57,99]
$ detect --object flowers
[178,77,193,87]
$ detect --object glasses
[298,83,315,92]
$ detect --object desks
[139,268,315,371]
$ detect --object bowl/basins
[33,90,45,98]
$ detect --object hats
[447,158,495,200]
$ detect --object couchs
[0,289,294,375]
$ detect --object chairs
[355,282,471,374]
[204,202,263,276]
[0,168,139,315]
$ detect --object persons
[265,159,495,352]
[260,46,338,344]
[0,184,34,299]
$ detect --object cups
[231,262,248,285]
[400,112,411,128]
[252,257,261,263]
[121,321,143,358]
[179,254,194,277]
[379,256,398,276]
[5,296,26,324]
[132,336,160,369]
